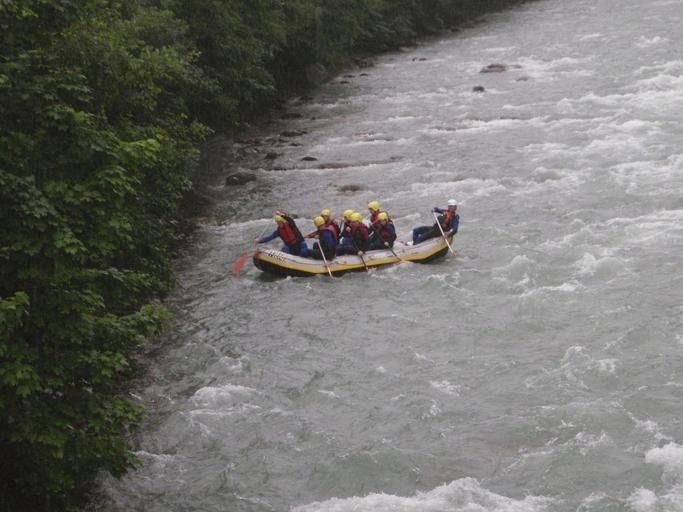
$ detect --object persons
[308,202,397,260]
[407,200,460,245]
[254,210,310,257]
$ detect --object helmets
[343,210,353,219]
[377,213,388,224]
[275,214,286,223]
[447,199,457,206]
[350,213,363,223]
[314,216,324,226]
[321,210,330,216]
[368,201,380,212]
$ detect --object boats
[250,226,452,280]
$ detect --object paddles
[232,216,276,272]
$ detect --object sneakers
[406,241,414,245]
